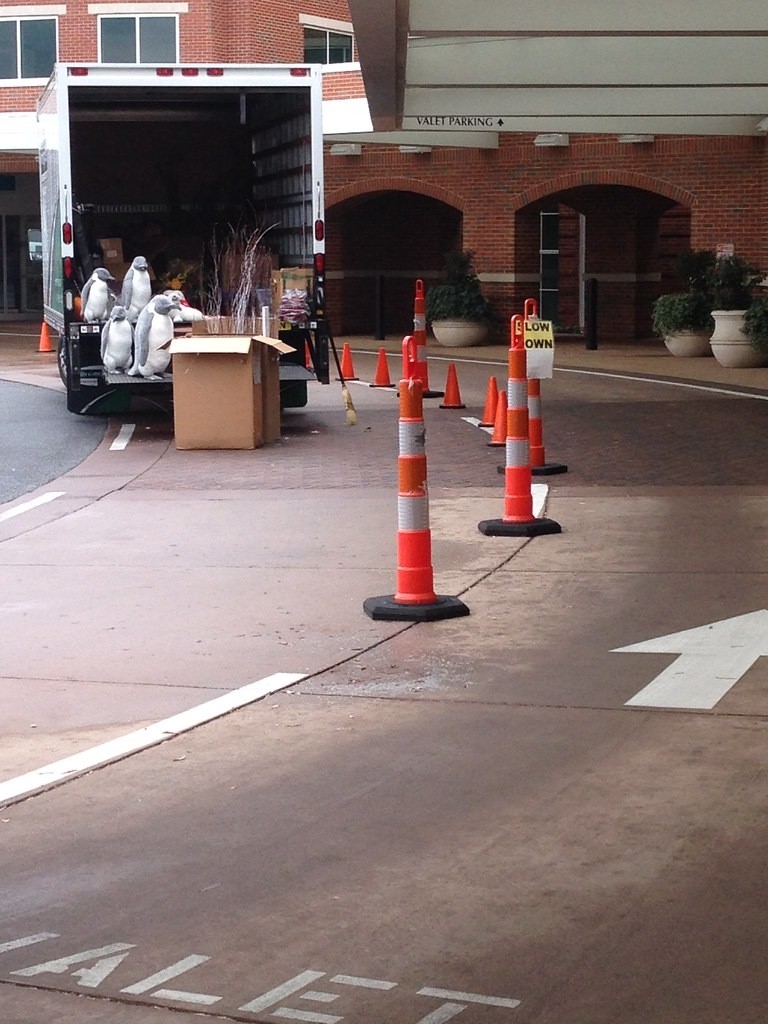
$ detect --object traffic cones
[439,361,467,409]
[369,348,395,387]
[477,376,507,447]
[35,321,56,352]
[335,343,359,380]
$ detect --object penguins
[122,256,151,323]
[81,268,115,323]
[101,306,135,374]
[128,295,181,380]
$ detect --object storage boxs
[224,286,269,317]
[221,254,278,290]
[104,263,156,283]
[156,337,297,449]
[97,237,124,263]
[192,317,279,447]
[269,267,313,317]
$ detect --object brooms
[316,289,358,427]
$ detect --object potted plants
[652,291,715,357]
[710,254,768,368]
[425,249,498,347]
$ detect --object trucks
[28,63,331,416]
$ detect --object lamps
[399,146,433,153]
[617,135,654,143]
[534,134,569,146]
[330,143,362,155]
